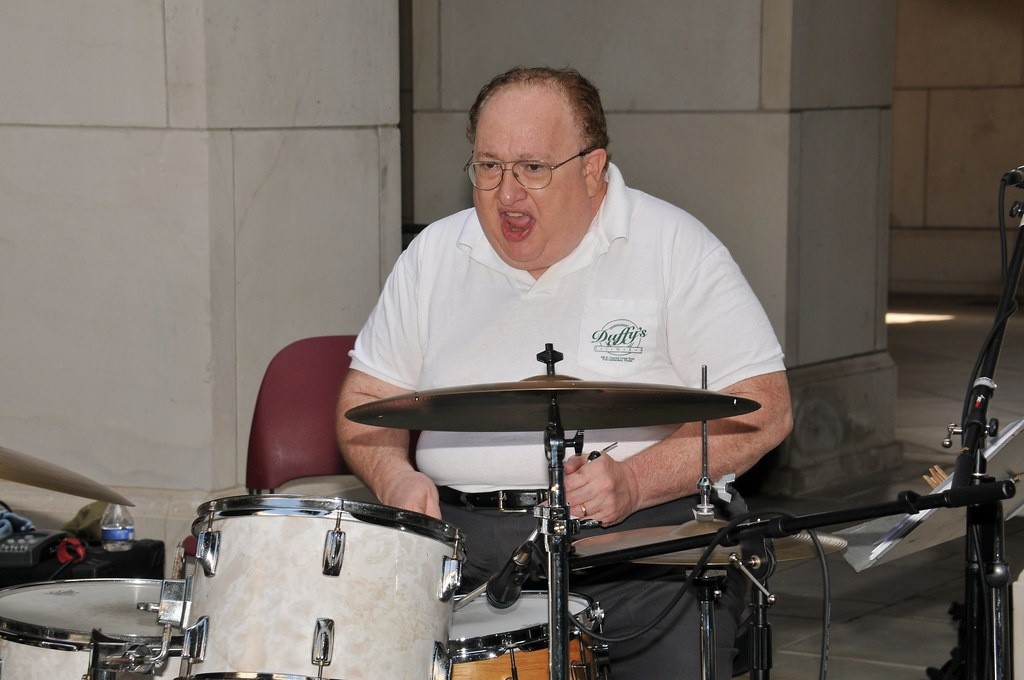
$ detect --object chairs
[246,336,422,495]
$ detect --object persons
[328,64,792,680]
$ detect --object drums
[173,485,472,680]
[444,586,614,680]
[0,572,204,680]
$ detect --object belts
[436,483,548,514]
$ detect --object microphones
[485,540,533,609]
[1001,166,1024,190]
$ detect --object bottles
[100,502,136,551]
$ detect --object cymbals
[0,441,142,512]
[562,512,851,572]
[340,370,767,440]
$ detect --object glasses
[464,145,606,190]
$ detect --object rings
[580,503,587,517]
